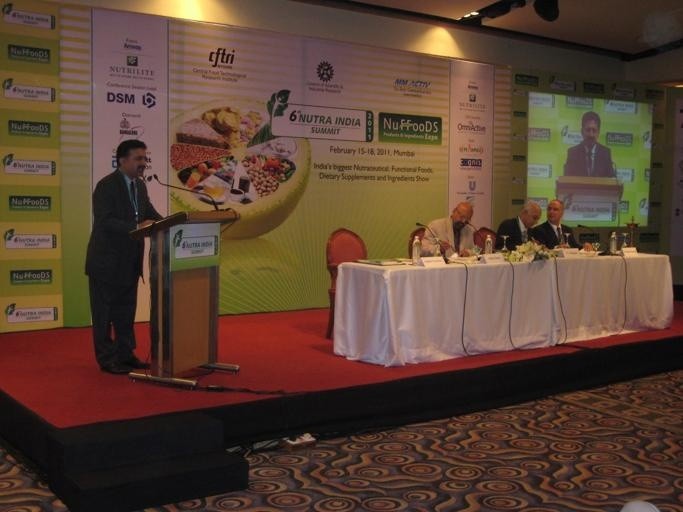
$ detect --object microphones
[153,174,230,211]
[462,219,503,262]
[415,220,453,264]
[577,225,614,256]
[526,229,555,259]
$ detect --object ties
[587,143,593,175]
[130,181,137,207]
[557,226,561,240]
[522,230,527,243]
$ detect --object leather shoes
[101,360,134,374]
[121,356,151,369]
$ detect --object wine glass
[564,233,573,257]
[500,235,510,259]
[592,240,600,257]
[621,231,630,254]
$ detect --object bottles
[412,236,422,265]
[484,235,493,259]
[610,232,618,256]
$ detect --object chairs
[325,226,497,339]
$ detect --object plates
[451,255,480,261]
[580,251,604,256]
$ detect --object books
[353,258,414,266]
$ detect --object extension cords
[279,434,317,446]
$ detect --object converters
[205,384,225,392]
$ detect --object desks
[332,250,674,367]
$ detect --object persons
[82,140,164,375]
[563,112,615,178]
[419,200,479,255]
[533,200,593,251]
[493,201,544,250]
